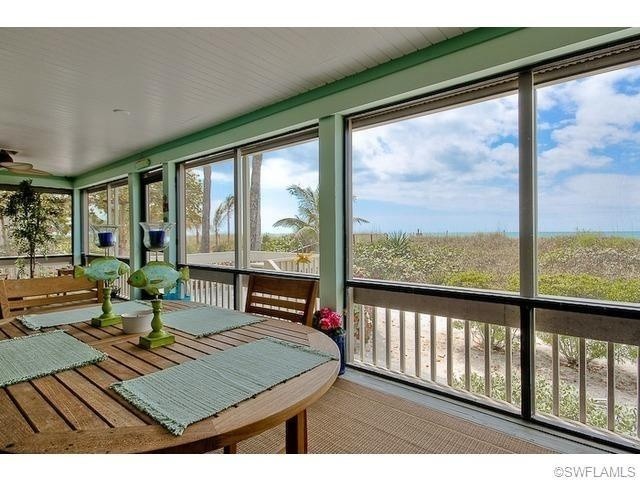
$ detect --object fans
[0,150,54,176]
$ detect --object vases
[331,336,345,376]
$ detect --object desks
[0,299,342,453]
[57,268,74,296]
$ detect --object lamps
[139,222,177,299]
[90,226,121,297]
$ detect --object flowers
[313,307,344,336]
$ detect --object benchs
[0,276,103,319]
[245,274,319,326]
